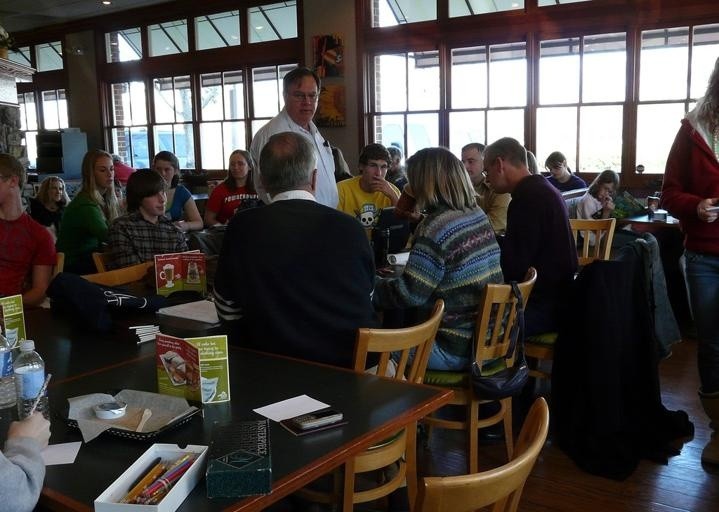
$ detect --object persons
[207,65,505,379]
[0,410,51,512]
[658,58,718,437]
[1,152,72,307]
[480,136,625,334]
[65,149,204,274]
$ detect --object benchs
[558,188,624,261]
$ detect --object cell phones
[294,409,344,431]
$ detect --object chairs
[418,268,537,476]
[37,252,65,309]
[298,298,446,512]
[412,396,550,512]
[93,252,112,273]
[568,218,617,266]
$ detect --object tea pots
[186,263,203,284]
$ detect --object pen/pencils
[119,452,196,504]
[25,373,53,420]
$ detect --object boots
[696,386,719,468]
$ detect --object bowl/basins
[653,212,667,221]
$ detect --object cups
[647,197,659,217]
[163,265,175,288]
[395,184,417,216]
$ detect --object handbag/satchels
[471,361,530,400]
[45,271,166,334]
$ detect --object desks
[0,344,453,512]
[0,306,204,386]
[620,205,681,235]
[192,193,209,203]
[111,259,224,337]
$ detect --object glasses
[287,91,319,99]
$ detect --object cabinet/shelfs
[36,132,89,180]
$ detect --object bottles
[14,340,49,423]
[0,326,17,410]
